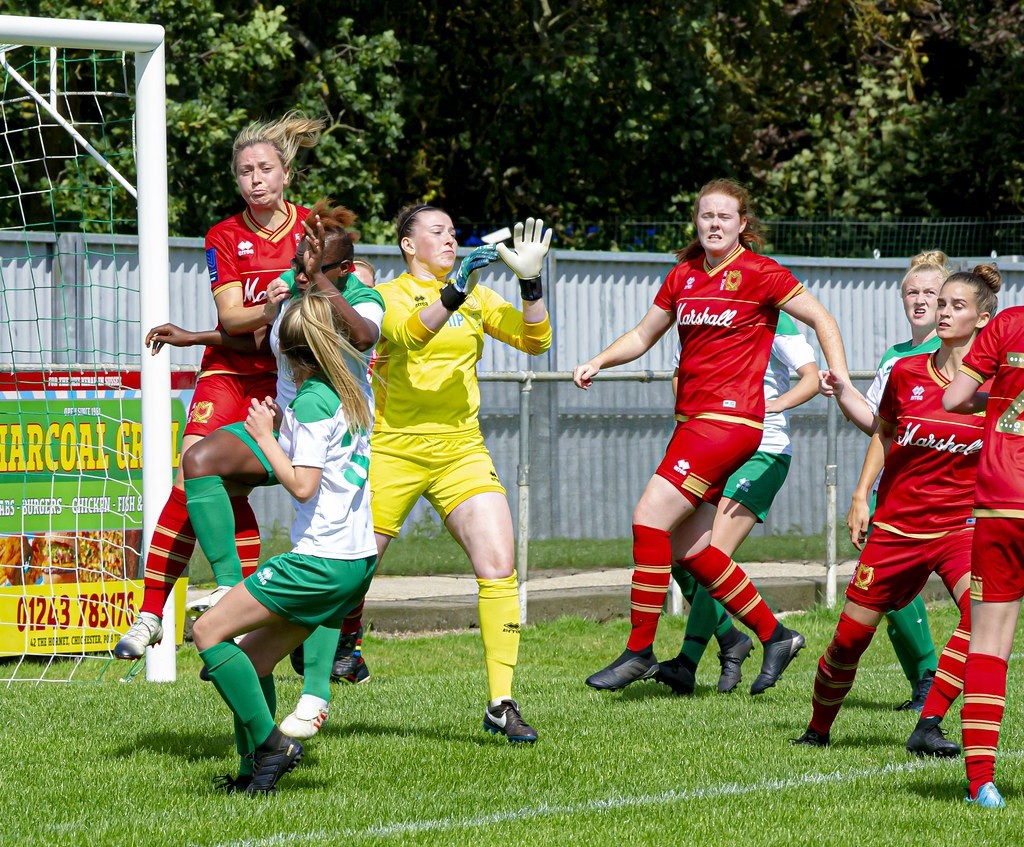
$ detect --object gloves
[495,217,552,280]
[450,244,499,296]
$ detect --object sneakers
[643,654,697,696]
[895,677,933,712]
[787,727,830,748]
[114,613,163,661]
[246,732,305,800]
[279,694,329,738]
[331,626,363,678]
[906,715,961,758]
[483,700,538,744]
[330,657,370,685]
[187,586,232,612]
[585,643,657,692]
[750,622,806,695]
[716,633,755,693]
[212,774,254,795]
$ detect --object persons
[941,303,1024,807]
[192,293,383,793]
[571,178,1001,756]
[371,204,553,744]
[112,119,387,793]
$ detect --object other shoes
[963,782,1006,810]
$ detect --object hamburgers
[42,532,82,583]
